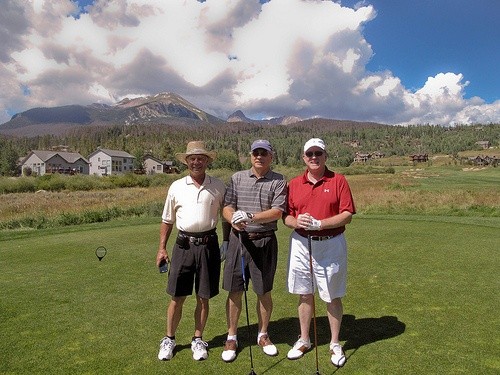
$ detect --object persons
[157,141,233,360]
[221,141,288,362]
[282,139,357,366]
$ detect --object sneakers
[191,338,208,360]
[158,336,176,361]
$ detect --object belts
[300,235,334,241]
[177,234,218,245]
[232,228,274,240]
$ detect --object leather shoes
[329,343,345,367]
[257,334,277,356]
[286,335,311,360]
[221,340,238,362]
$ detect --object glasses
[251,151,271,157]
[305,151,325,157]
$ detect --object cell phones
[159,259,168,273]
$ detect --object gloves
[231,208,255,224]
[304,216,321,230]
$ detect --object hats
[251,139,273,152]
[304,138,325,154]
[175,140,217,165]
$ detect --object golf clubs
[238,232,257,375]
[306,230,321,375]
